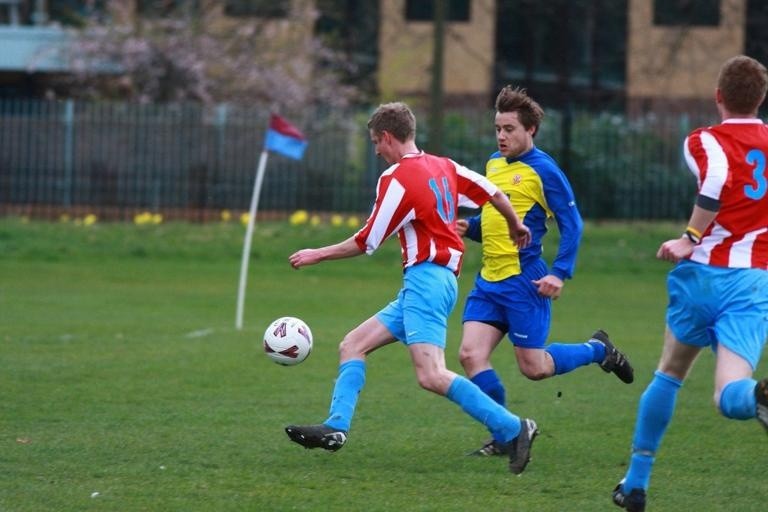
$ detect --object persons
[452,85,636,458]
[610,54,768,512]
[283,100,541,476]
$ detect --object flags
[264,112,311,160]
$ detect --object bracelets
[681,226,704,244]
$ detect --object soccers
[263,316,312,367]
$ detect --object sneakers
[755,378,768,431]
[509,418,538,475]
[612,479,647,511]
[592,329,634,384]
[285,426,348,452]
[468,440,510,456]
